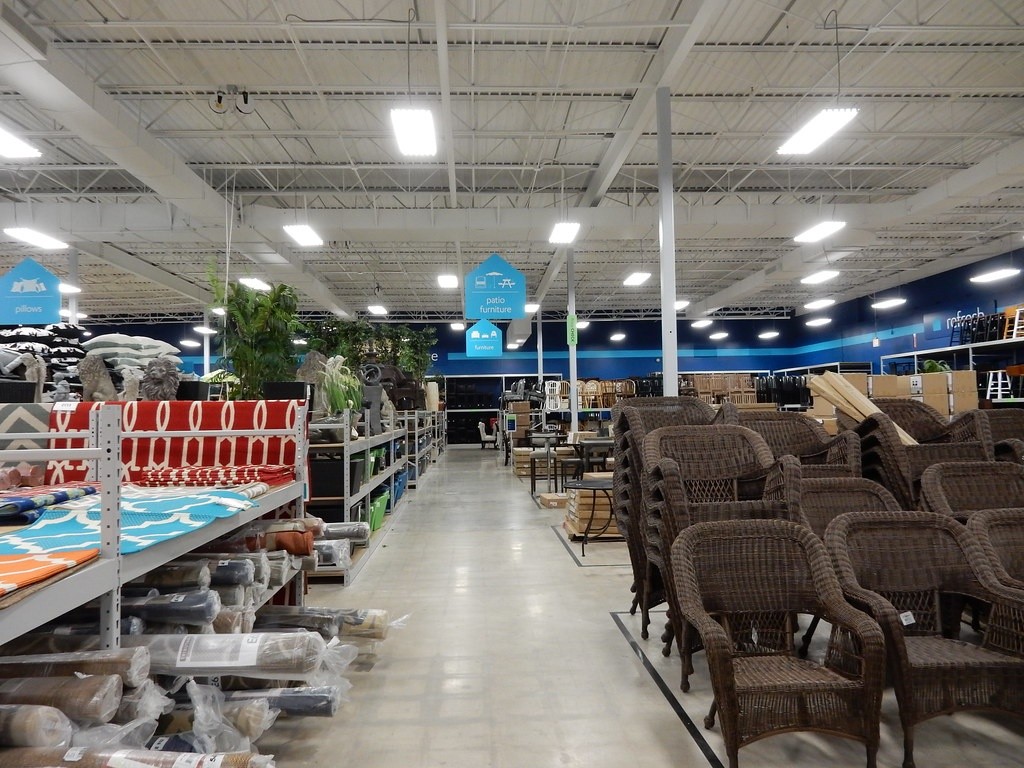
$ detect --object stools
[529,449,558,494]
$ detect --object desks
[579,439,613,471]
[527,434,568,492]
[564,480,612,555]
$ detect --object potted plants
[206,257,447,428]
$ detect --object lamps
[969,268,1021,283]
[282,224,324,248]
[0,127,91,337]
[389,109,438,156]
[507,108,907,351]
[367,305,388,315]
[437,275,458,289]
[450,323,464,330]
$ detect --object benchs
[560,456,605,491]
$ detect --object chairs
[948,308,1024,347]
[379,365,420,410]
[611,397,1024,768]
[1006,364,1024,399]
[976,371,990,399]
[477,378,636,557]
[986,370,1014,401]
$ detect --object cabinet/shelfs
[397,410,433,490]
[431,411,446,463]
[0,398,310,767]
[307,409,409,556]
[772,361,874,406]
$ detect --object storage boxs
[687,370,979,437]
[505,425,525,438]
[507,413,530,426]
[508,401,530,414]
